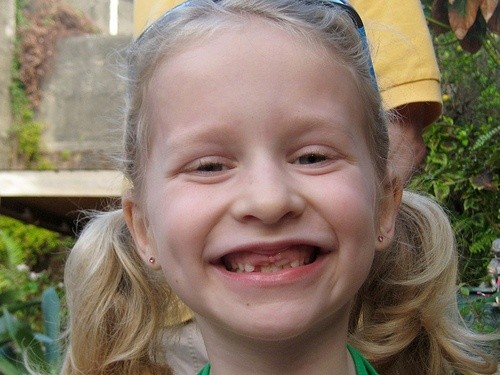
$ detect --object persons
[133,1,443,186]
[24,0,500,375]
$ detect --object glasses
[132,0,385,118]
